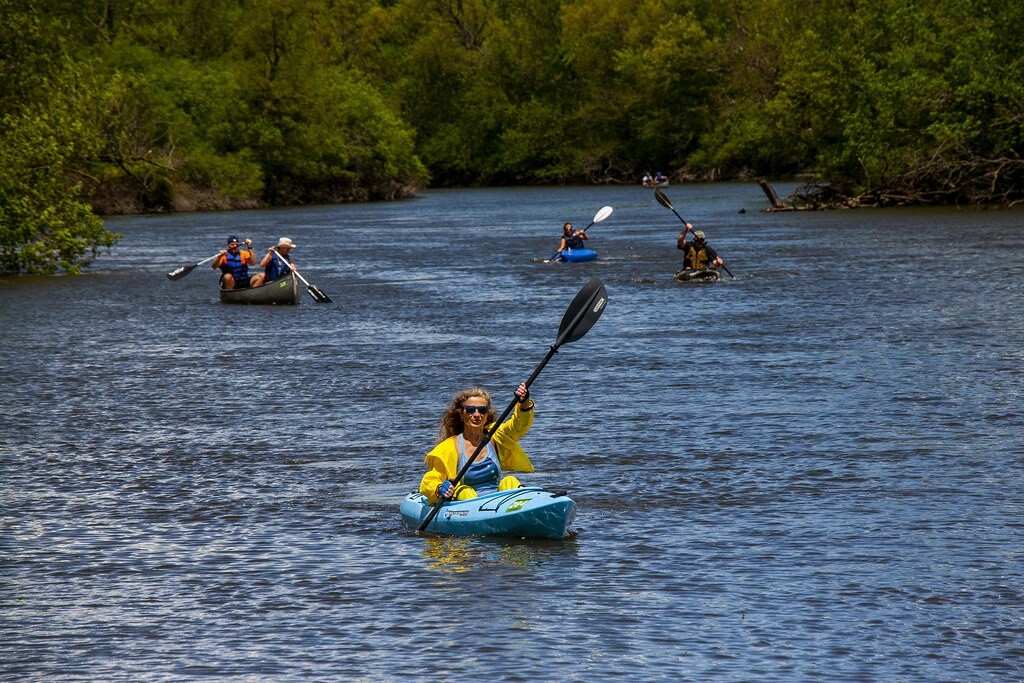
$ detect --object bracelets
[248,245,253,251]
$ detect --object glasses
[566,228,572,230]
[464,406,490,415]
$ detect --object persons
[211,236,264,289]
[260,237,297,285]
[419,383,534,506]
[558,223,588,252]
[642,171,667,185]
[678,223,723,271]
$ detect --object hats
[693,231,705,240]
[227,236,239,245]
[278,237,297,248]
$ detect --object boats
[399,489,578,539]
[674,269,720,283]
[645,180,669,188]
[558,248,597,263]
[219,272,300,305]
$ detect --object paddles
[273,247,334,303]
[167,240,249,282]
[654,188,737,280]
[543,205,614,262]
[418,277,609,531]
